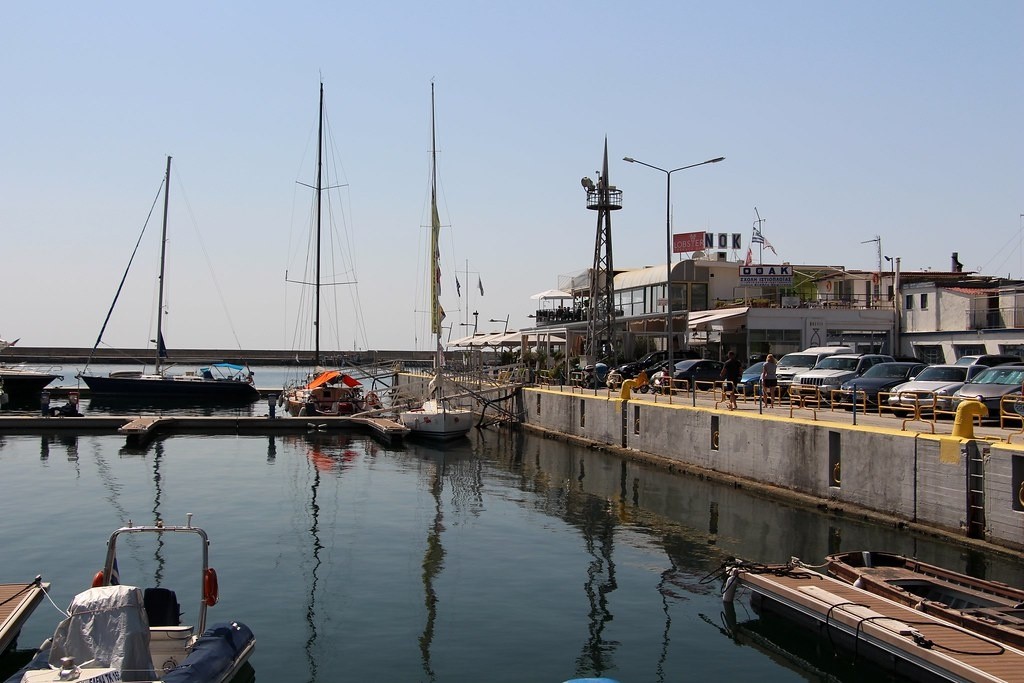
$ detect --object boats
[4,513,256,683]
[698,550,1023,683]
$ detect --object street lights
[460,322,477,352]
[528,313,549,349]
[623,156,725,394]
[489,314,509,360]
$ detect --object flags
[763,237,777,256]
[479,278,484,296]
[456,277,461,297]
[752,227,764,244]
[746,248,752,266]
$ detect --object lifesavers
[92,569,104,587]
[826,281,832,292]
[204,568,219,606]
[873,274,879,284]
[365,393,378,405]
[411,408,426,412]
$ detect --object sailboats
[398,82,475,444]
[278,83,364,417]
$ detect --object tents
[530,289,575,310]
[446,332,566,352]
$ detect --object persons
[762,354,778,409]
[652,364,676,395]
[531,367,536,383]
[609,367,618,384]
[560,368,566,385]
[538,306,581,320]
[719,351,744,411]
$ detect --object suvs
[619,350,702,380]
[790,353,897,407]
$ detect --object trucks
[759,347,854,404]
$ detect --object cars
[722,362,765,399]
[643,358,686,380]
[840,355,1024,418]
[649,359,723,394]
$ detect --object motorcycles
[631,368,649,393]
[76,157,261,397]
[648,363,681,395]
